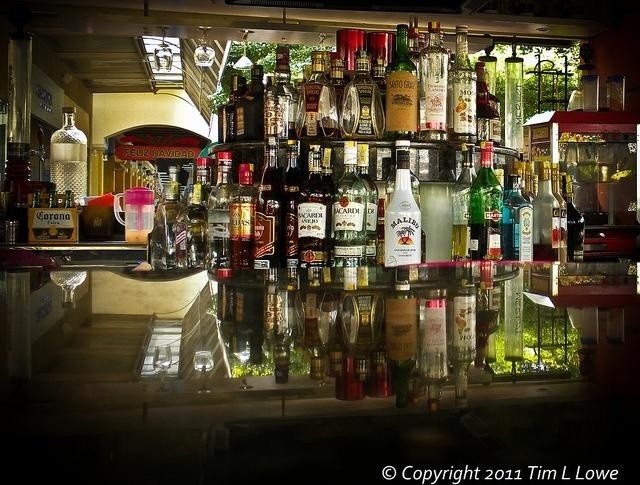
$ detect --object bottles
[223,74,255,141]
[384,150,422,267]
[338,50,386,140]
[32,190,40,209]
[295,287,336,380]
[476,285,498,368]
[155,182,186,269]
[353,144,377,269]
[298,144,330,268]
[285,140,299,259]
[386,23,419,140]
[262,285,295,384]
[218,76,233,145]
[205,152,233,275]
[318,60,348,139]
[63,189,75,234]
[182,157,213,206]
[448,287,477,406]
[419,290,448,413]
[254,140,288,271]
[533,162,561,262]
[420,21,449,141]
[48,106,88,203]
[48,271,88,309]
[304,51,331,138]
[333,139,369,283]
[452,143,477,259]
[337,291,385,383]
[552,168,569,263]
[229,164,257,270]
[384,140,421,209]
[502,174,533,262]
[384,291,418,406]
[318,148,336,208]
[185,183,208,265]
[468,141,501,261]
[562,175,585,262]
[243,285,263,365]
[449,25,477,142]
[327,317,344,379]
[474,61,501,143]
[247,64,265,107]
[265,46,303,142]
[216,281,231,323]
[155,164,182,209]
[363,359,391,400]
[47,191,58,237]
[372,56,387,113]
[229,285,246,352]
[334,351,362,402]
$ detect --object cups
[113,187,154,245]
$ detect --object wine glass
[193,24,216,68]
[192,351,215,395]
[154,24,174,71]
[151,345,173,395]
[232,29,255,70]
[235,350,251,391]
